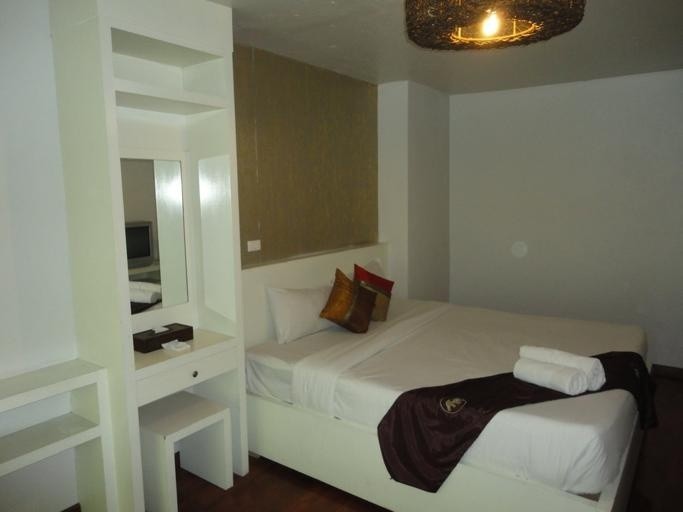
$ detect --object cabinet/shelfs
[0,1,250,512]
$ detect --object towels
[513,357,587,396]
[128,281,162,304]
[518,345,607,393]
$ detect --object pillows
[266,285,338,344]
[320,267,378,333]
[352,263,394,321]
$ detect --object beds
[241,243,653,512]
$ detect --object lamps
[404,0,586,51]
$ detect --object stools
[139,391,234,512]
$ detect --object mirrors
[119,157,188,315]
